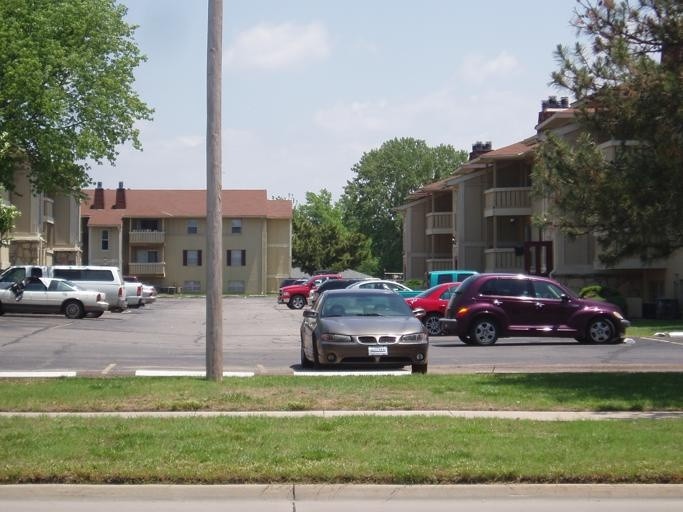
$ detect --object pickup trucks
[397,270,479,301]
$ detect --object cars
[438,272,631,348]
[0,259,158,319]
[403,282,462,337]
[296,286,430,376]
[274,266,413,310]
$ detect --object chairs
[325,302,390,319]
